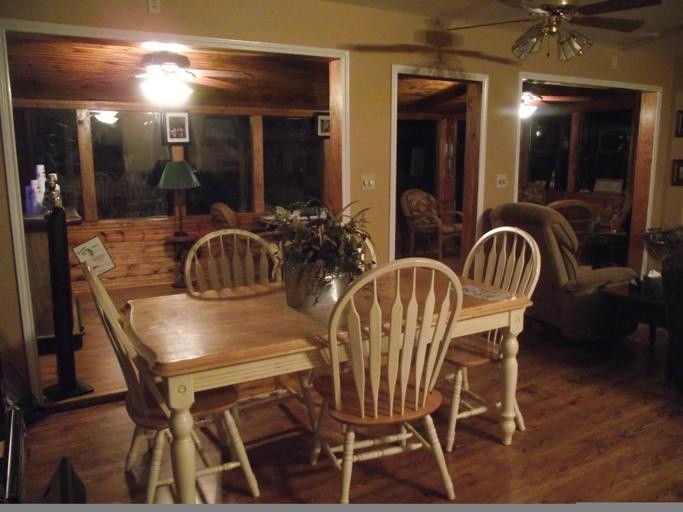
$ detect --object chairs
[309,256,456,504]
[79,260,261,504]
[484,203,640,341]
[399,189,463,260]
[425,226,526,453]
[185,228,316,433]
[211,203,276,261]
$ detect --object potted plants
[263,196,376,308]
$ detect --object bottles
[22,163,64,216]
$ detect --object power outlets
[147,0,161,14]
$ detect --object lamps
[156,160,203,236]
[132,50,196,107]
[511,20,592,62]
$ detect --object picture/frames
[670,159,682,186]
[162,112,192,146]
[675,111,682,138]
[313,112,330,138]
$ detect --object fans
[444,0,662,31]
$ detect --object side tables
[595,280,683,373]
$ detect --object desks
[121,259,534,505]
